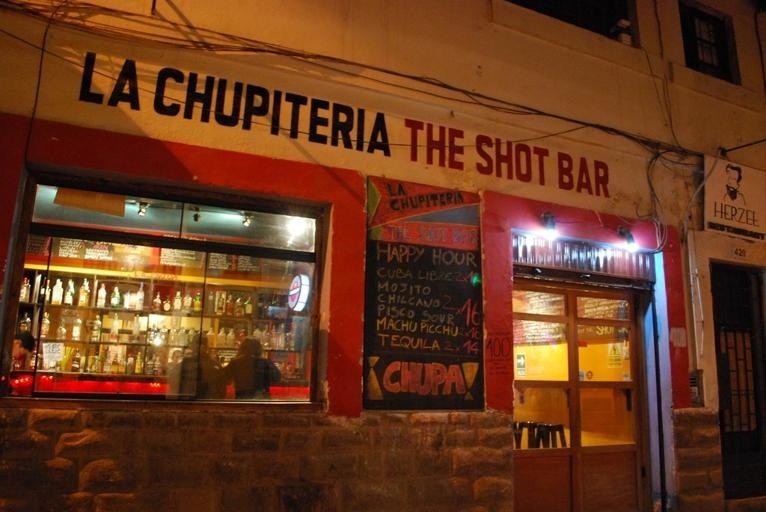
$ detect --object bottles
[10,274,309,382]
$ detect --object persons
[165,350,182,376]
[165,331,227,402]
[10,329,35,360]
[181,345,193,357]
[219,336,282,400]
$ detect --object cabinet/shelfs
[11,262,312,388]
